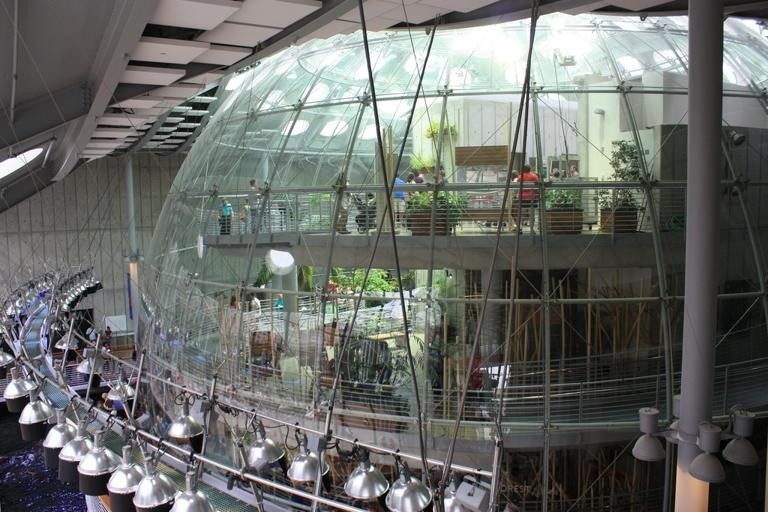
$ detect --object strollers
[352,194,377,232]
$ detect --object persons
[248,179,262,234]
[250,292,260,311]
[216,198,234,235]
[274,294,284,310]
[102,326,113,339]
[337,168,425,234]
[231,295,240,311]
[244,199,250,235]
[86,323,96,342]
[511,162,579,230]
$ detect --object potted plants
[593,138,657,236]
[426,120,456,142]
[537,183,585,235]
[405,155,445,175]
[403,192,471,238]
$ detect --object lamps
[730,128,745,146]
[630,395,761,485]
